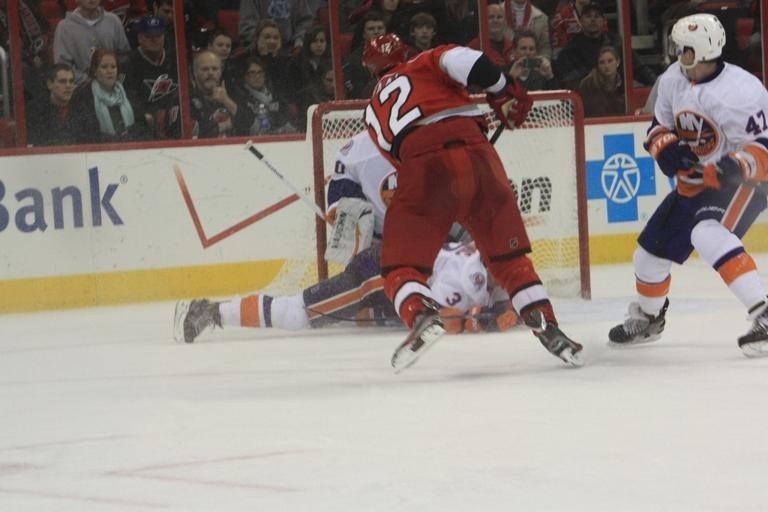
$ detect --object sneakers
[184,298,223,342]
[536,325,583,360]
[609,297,669,343]
[738,295,768,348]
[391,312,444,367]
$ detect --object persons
[320,128,478,267]
[609,11,767,344]
[184,233,537,343]
[1,1,768,142]
[361,30,587,371]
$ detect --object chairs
[31,1,237,65]
[630,1,766,111]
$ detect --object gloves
[716,152,743,189]
[485,76,533,130]
[657,140,699,179]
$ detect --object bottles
[256,105,272,136]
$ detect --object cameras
[523,57,542,67]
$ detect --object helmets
[668,13,726,69]
[362,32,407,75]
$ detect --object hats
[138,16,166,33]
